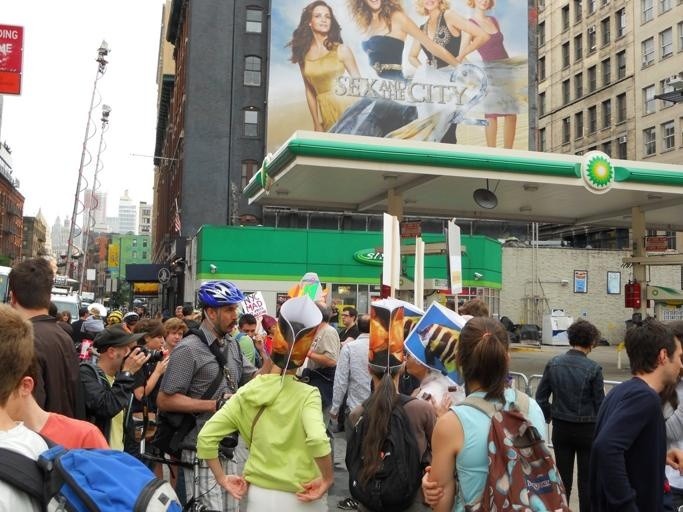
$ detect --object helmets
[196,279,244,307]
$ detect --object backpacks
[454,389,572,512]
[0,430,184,512]
[344,390,429,512]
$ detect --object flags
[174,202,182,234]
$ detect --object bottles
[421,391,431,402]
[446,386,464,408]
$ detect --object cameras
[127,345,164,368]
[215,394,239,450]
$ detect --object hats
[93,323,148,353]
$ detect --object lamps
[475,272,483,280]
[210,264,217,274]
[473,179,501,209]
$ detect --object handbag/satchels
[303,366,336,407]
[153,408,196,460]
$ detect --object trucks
[49,284,81,325]
[80,291,94,310]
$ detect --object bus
[0,266,13,303]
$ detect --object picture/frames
[607,270,622,295]
[574,269,588,294]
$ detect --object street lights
[78,102,111,289]
[63,36,110,279]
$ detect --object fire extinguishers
[625,280,632,307]
[633,279,641,309]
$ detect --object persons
[461,1,520,149]
[283,0,365,131]
[328,0,462,139]
[407,0,493,145]
[1,260,683,512]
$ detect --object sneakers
[337,496,358,510]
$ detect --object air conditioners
[619,136,628,144]
[588,26,595,34]
